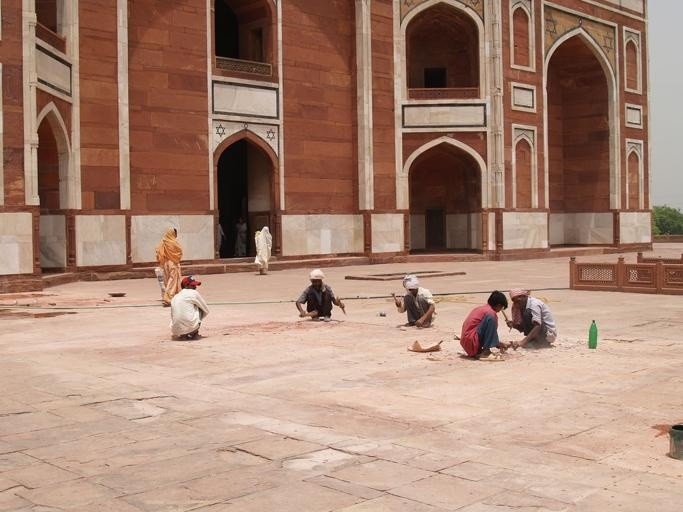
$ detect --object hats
[181,276,201,289]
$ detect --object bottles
[588,319,598,349]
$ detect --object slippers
[479,352,505,361]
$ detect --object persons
[255,227,272,275]
[169,277,210,341]
[459,290,508,361]
[295,269,345,322]
[504,288,557,350]
[218,223,226,255]
[155,228,183,307]
[234,216,248,257]
[394,275,435,328]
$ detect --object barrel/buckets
[669,423,681,458]
[155,265,165,296]
